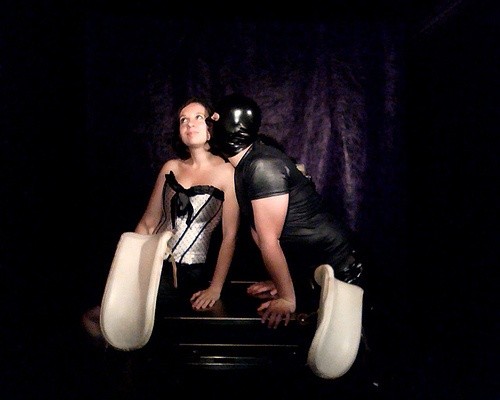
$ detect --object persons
[81,98,239,353]
[212,93,376,399]
